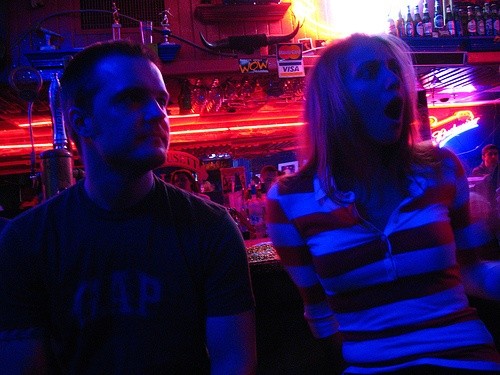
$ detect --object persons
[1,40,500,375]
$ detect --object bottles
[434,0,444,32]
[405,5,414,37]
[454,3,499,35]
[421,0,432,37]
[387,13,397,37]
[445,5,456,37]
[413,4,424,38]
[397,7,405,37]
